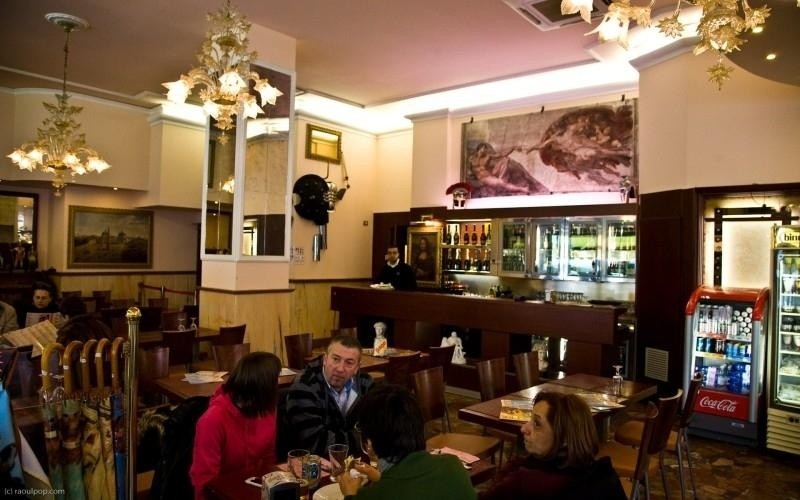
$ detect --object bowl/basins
[442,279,465,295]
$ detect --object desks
[548,373,660,403]
[459,382,629,476]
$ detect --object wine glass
[189,317,197,332]
[611,364,624,396]
[177,317,185,333]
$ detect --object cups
[285,422,379,500]
[361,347,398,356]
[550,291,583,306]
[782,335,800,347]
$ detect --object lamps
[559,0,778,93]
[158,0,286,138]
[8,11,117,198]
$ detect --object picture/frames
[67,204,155,270]
[405,225,442,291]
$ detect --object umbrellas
[40,337,124,500]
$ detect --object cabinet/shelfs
[499,220,566,281]
[442,222,497,276]
[567,218,635,282]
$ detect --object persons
[412,237,434,281]
[188,352,281,500]
[339,383,480,500]
[464,105,634,196]
[374,245,418,292]
[284,335,375,458]
[478,391,629,500]
[372,320,388,358]
[1,284,118,391]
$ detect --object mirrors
[236,62,296,266]
[304,123,342,165]
[199,63,234,263]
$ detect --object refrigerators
[680,284,768,450]
[766,222,800,457]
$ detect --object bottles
[694,362,750,394]
[444,222,491,272]
[373,322,388,357]
[487,283,512,299]
[502,227,636,275]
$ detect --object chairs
[476,355,522,458]
[513,351,542,392]
[613,371,705,500]
[0,287,505,499]
[592,400,660,497]
